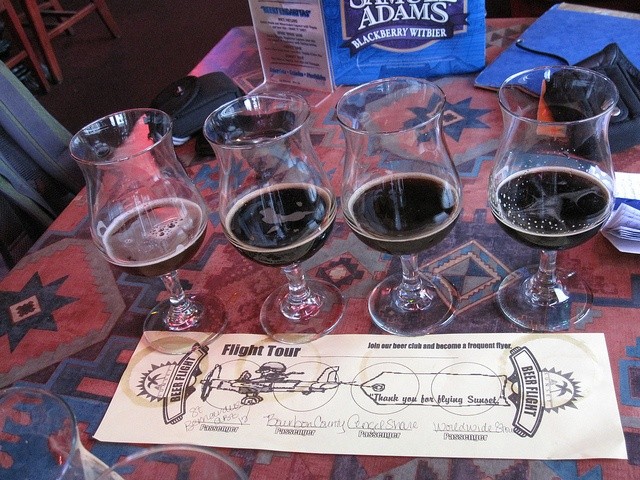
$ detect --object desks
[0,15,640,479]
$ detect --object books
[601,172,640,255]
[473,2,639,92]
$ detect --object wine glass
[67,109,230,353]
[487,65,619,333]
[337,76,462,336]
[204,93,345,346]
